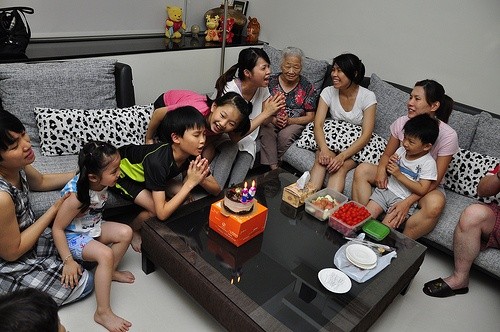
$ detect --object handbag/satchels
[0,6,34,56]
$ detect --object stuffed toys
[204,15,234,43]
[165,6,186,38]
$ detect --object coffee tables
[140,168,430,332]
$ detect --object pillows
[38,103,160,154]
[439,147,500,206]
[296,115,389,169]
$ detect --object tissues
[281,171,319,209]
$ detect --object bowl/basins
[303,187,372,236]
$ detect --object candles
[248,179,257,199]
[242,181,249,202]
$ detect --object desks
[0,34,271,102]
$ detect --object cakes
[223,187,254,214]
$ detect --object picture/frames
[231,0,249,39]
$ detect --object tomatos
[329,202,371,236]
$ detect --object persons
[52,140,135,332]
[0,288,66,332]
[146,90,251,162]
[212,47,286,189]
[365,113,439,229]
[423,166,500,297]
[108,105,220,252]
[352,79,458,239]
[310,53,377,192]
[0,109,94,305]
[259,47,316,170]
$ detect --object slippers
[423,278,468,297]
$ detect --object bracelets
[63,253,72,263]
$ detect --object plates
[317,268,352,294]
[346,243,378,270]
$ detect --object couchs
[0,57,164,221]
[248,42,500,280]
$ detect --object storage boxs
[328,199,375,237]
[305,186,348,223]
[208,197,269,247]
